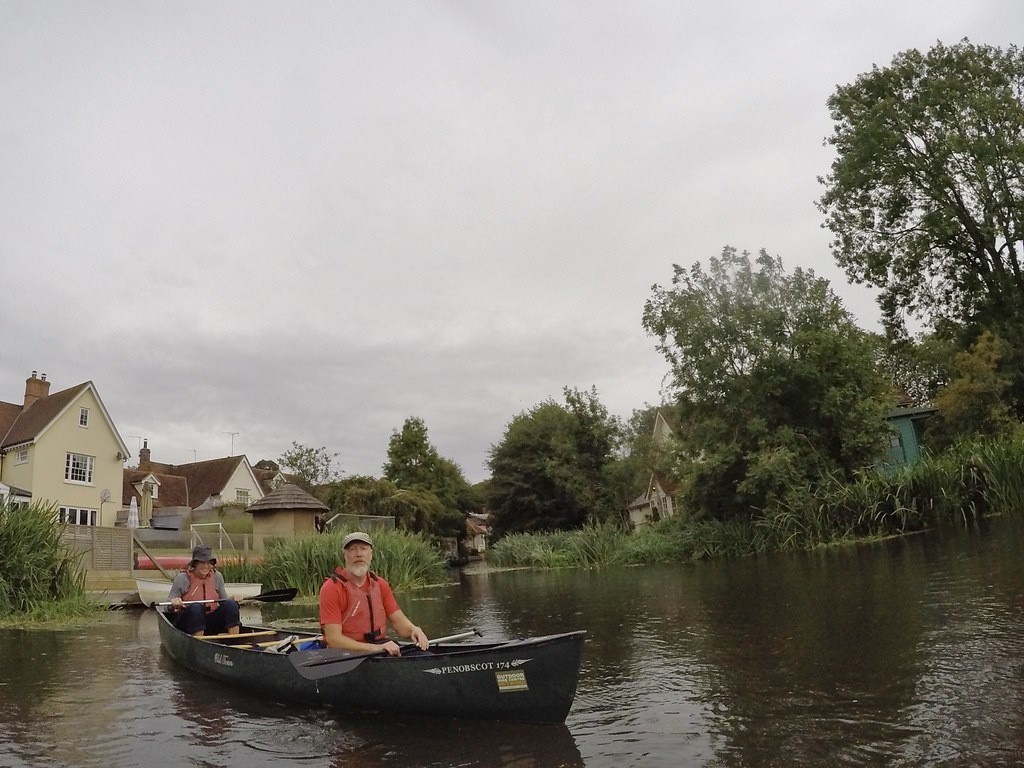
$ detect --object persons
[167,545,241,645]
[320,531,429,656]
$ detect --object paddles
[288,626,484,681]
[92,587,299,611]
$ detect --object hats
[188,545,217,565]
[342,532,374,548]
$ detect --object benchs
[234,636,324,648]
[194,630,276,640]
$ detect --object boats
[154,603,587,726]
[133,577,263,607]
